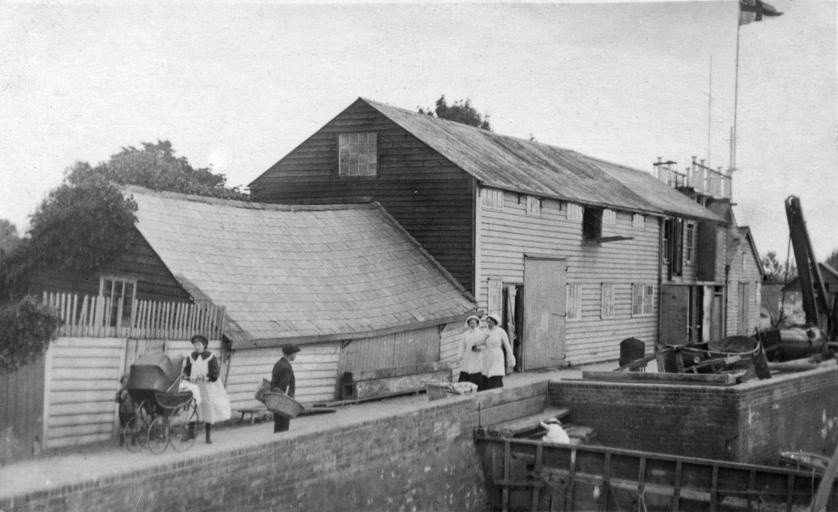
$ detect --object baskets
[254,378,305,419]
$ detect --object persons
[115,375,136,447]
[458,314,516,391]
[540,417,569,444]
[272,343,301,432]
[180,335,220,444]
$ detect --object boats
[471,194,838,512]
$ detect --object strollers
[113,350,199,457]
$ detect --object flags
[739,0,784,25]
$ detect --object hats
[191,333,207,348]
[466,316,480,328]
[282,343,301,354]
[487,314,500,326]
[544,418,561,425]
